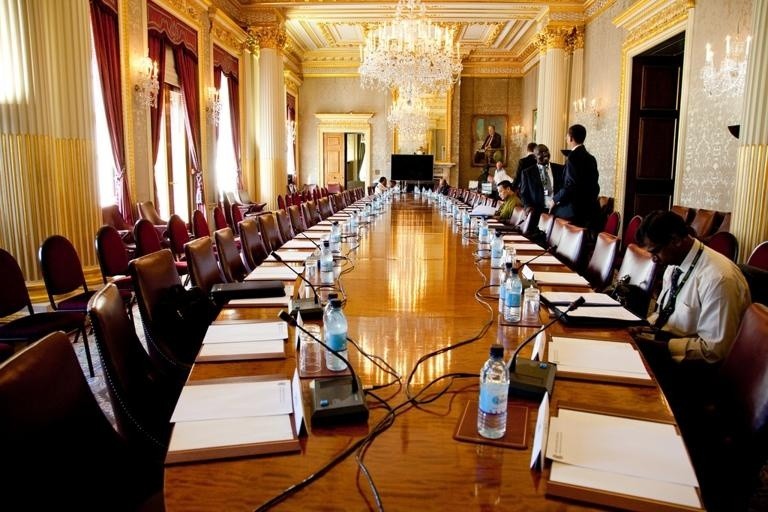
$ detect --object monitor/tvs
[391,154,433,181]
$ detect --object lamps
[132,56,160,109]
[510,125,527,148]
[205,87,220,127]
[573,98,607,131]
[701,34,751,101]
[357,1,463,155]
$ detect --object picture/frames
[532,109,538,147]
[472,114,508,166]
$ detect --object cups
[304,260,319,285]
[300,324,321,373]
[522,288,540,323]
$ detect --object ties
[540,165,552,197]
[485,135,492,147]
[652,267,684,331]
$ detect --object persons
[487,124,602,260]
[437,178,451,195]
[475,126,501,163]
[374,177,389,195]
[629,210,752,363]
[389,180,401,194]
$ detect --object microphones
[277,309,370,426]
[506,296,586,401]
[458,199,472,207]
[517,244,558,295]
[469,202,482,214]
[503,220,524,236]
[334,186,382,216]
[317,211,333,224]
[296,229,336,267]
[271,251,324,319]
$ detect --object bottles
[422,187,522,440]
[320,184,401,372]
